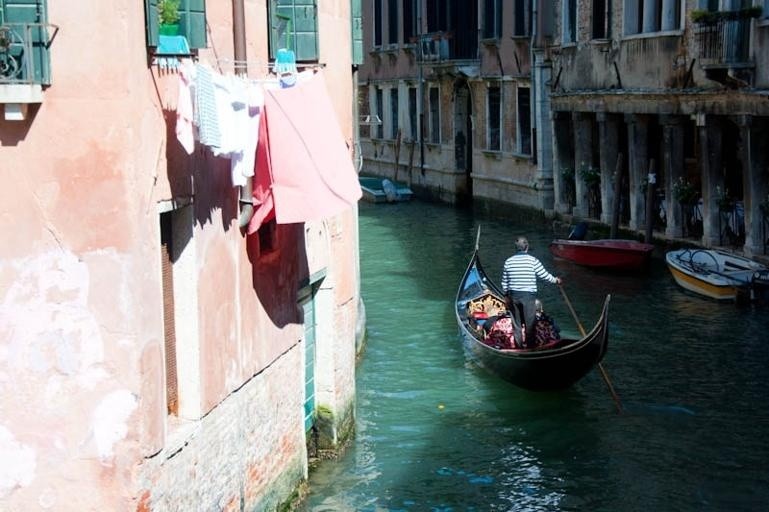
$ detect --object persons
[534,298,560,346]
[500,235,564,348]
[485,308,525,349]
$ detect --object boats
[454,223,612,392]
[359,176,414,204]
[548,238,658,268]
[665,248,769,303]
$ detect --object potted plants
[155,0,181,35]
[690,8,710,27]
